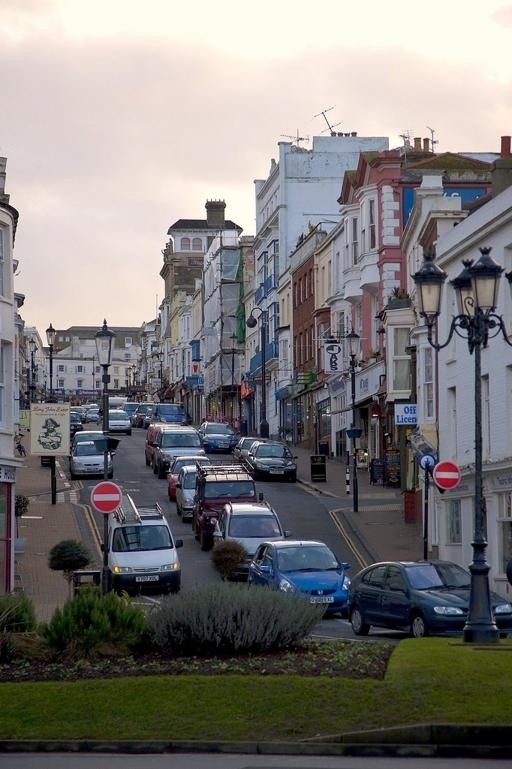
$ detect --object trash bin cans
[310,455,326,482]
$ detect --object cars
[248,538,350,619]
[69,396,299,523]
[213,501,291,581]
[345,561,511,640]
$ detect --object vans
[102,506,183,596]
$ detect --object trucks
[193,461,264,551]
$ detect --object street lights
[125,364,140,395]
[158,349,164,402]
[46,323,60,506]
[245,308,269,438]
[417,450,437,566]
[345,325,360,513]
[407,241,512,643]
[94,316,116,598]
[24,336,45,414]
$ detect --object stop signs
[89,480,123,514]
[433,458,462,491]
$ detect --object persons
[98,406,103,417]
[39,418,62,450]
